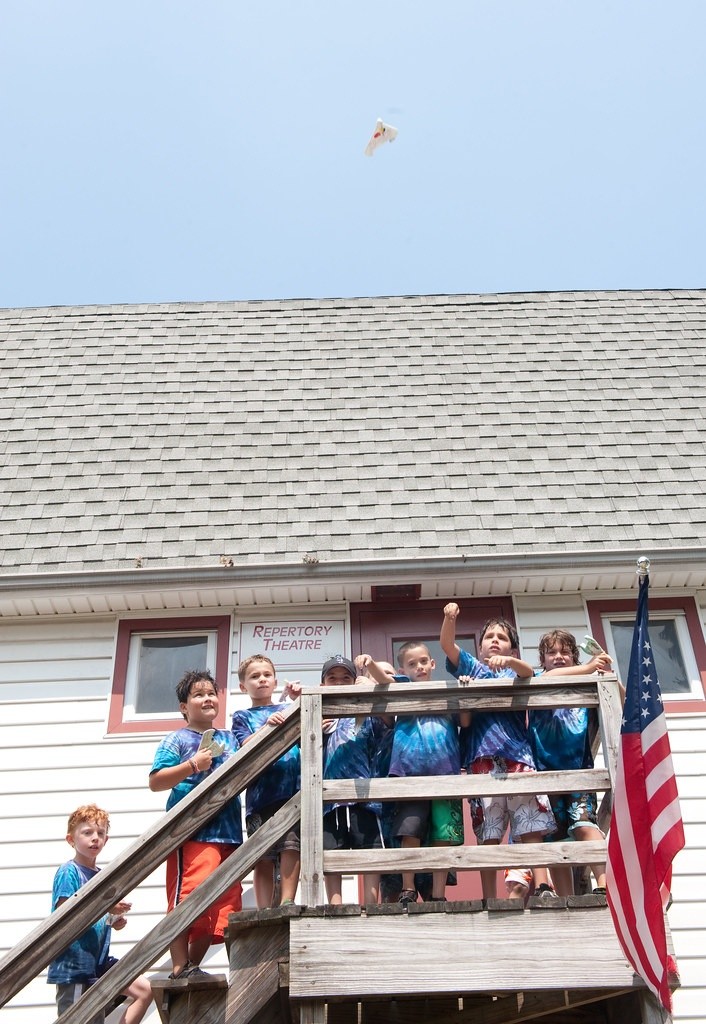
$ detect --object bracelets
[189,758,200,774]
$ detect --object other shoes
[534,883,558,897]
[396,889,418,907]
[168,960,208,978]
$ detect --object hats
[321,654,357,679]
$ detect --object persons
[232,653,304,911]
[359,661,460,902]
[317,653,383,907]
[439,602,557,906]
[46,803,154,1024]
[353,639,469,904]
[147,666,244,979]
[506,823,557,899]
[522,627,628,899]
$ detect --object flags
[605,579,688,1015]
